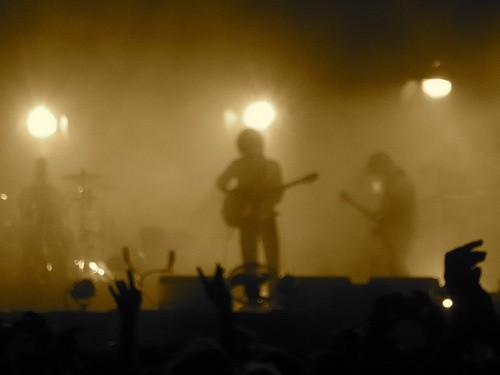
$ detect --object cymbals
[62,174,101,180]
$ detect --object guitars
[338,191,377,222]
[224,171,317,228]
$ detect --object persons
[366,151,416,278]
[1,238,499,375]
[216,128,284,311]
[17,159,75,288]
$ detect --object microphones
[168,250,176,266]
[122,245,131,265]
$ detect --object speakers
[365,276,439,306]
[279,275,352,308]
[159,275,232,309]
